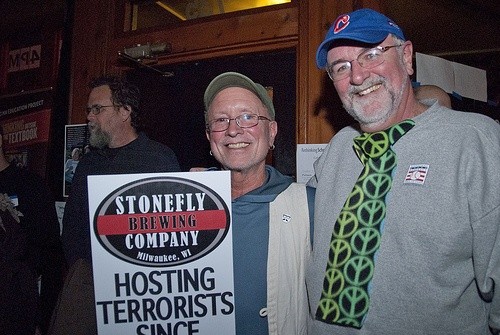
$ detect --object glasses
[325,45,401,81]
[206,114,270,132]
[87,105,119,115]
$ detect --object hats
[316,8,405,70]
[204,72,275,121]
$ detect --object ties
[315,119,416,328]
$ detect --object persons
[189,9,500,335]
[60,74,182,263]
[413,84,451,110]
[203,72,316,335]
[0,123,60,335]
